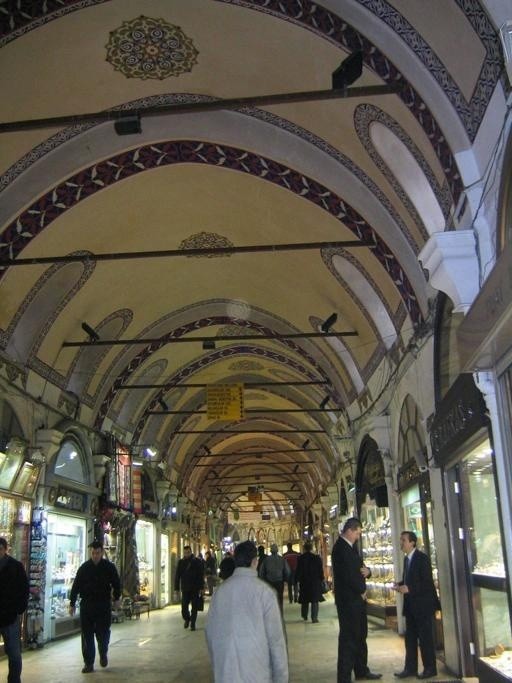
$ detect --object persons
[393,530,442,678]
[172,536,332,631]
[69,542,121,672]
[0,537,28,681]
[332,517,383,682]
[204,541,289,682]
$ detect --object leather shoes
[356,672,381,679]
[82,656,107,673]
[394,670,436,678]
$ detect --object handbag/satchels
[197,592,204,611]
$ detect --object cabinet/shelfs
[46,509,85,639]
[456,451,512,682]
[360,503,397,628]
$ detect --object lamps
[121,442,158,456]
[99,451,143,467]
